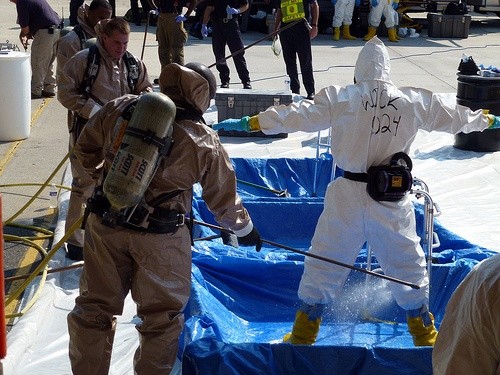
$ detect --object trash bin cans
[455,66,500,152]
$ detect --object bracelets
[310,23,318,27]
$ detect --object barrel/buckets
[453,71,500,153]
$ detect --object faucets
[411,176,429,252]
[409,189,434,277]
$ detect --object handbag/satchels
[367,152,412,201]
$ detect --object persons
[68,0,402,43]
[55,0,113,86]
[210,35,500,346]
[57,17,154,261]
[67,62,263,375]
[431,252,500,375]
[270,0,320,99]
[200,0,252,89]
[147,0,198,85]
[10,0,63,98]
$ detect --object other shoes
[407,312,438,346]
[244,83,251,89]
[31,93,41,99]
[307,92,314,99]
[67,244,83,260]
[154,79,159,84]
[42,90,55,97]
[283,310,320,345]
[221,82,229,87]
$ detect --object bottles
[462,52,468,63]
[284,75,290,94]
[49,183,57,208]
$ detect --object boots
[343,25,356,40]
[388,28,399,42]
[394,28,401,40]
[363,27,376,40]
[334,27,340,40]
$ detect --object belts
[342,171,367,181]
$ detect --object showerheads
[272,189,281,194]
[410,284,420,290]
[149,10,156,15]
[302,17,313,31]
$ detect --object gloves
[236,227,262,252]
[212,116,251,133]
[486,115,500,129]
[201,24,208,39]
[175,15,186,23]
[226,5,240,15]
